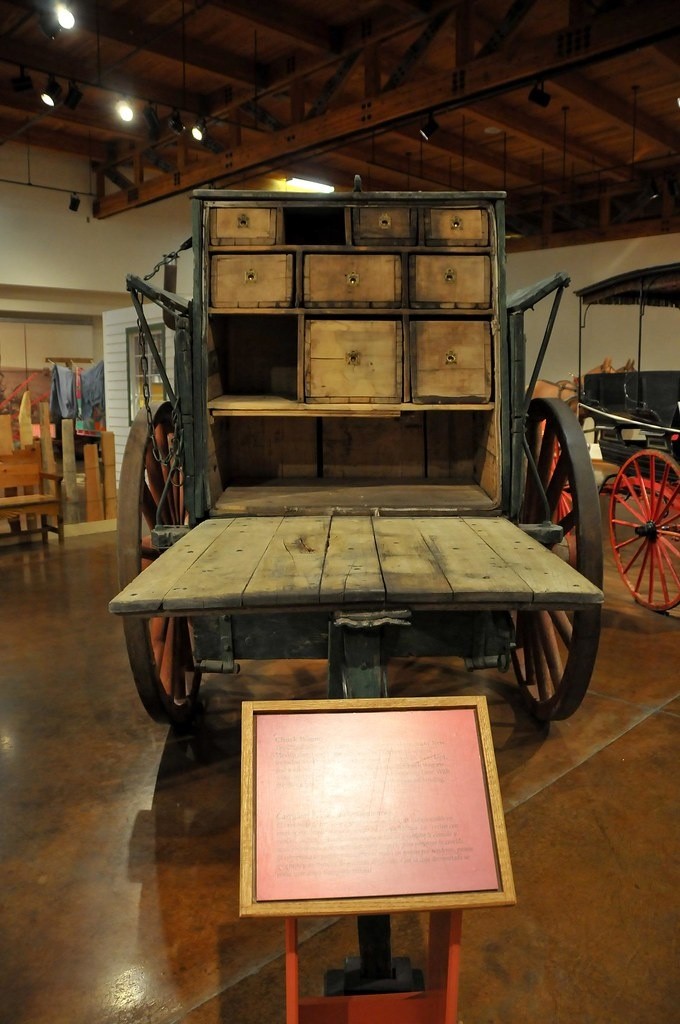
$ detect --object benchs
[583,370,679,454]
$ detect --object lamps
[69,193,81,212]
[192,117,207,141]
[420,112,439,141]
[143,107,161,130]
[167,111,186,137]
[528,81,552,108]
[39,13,62,40]
[11,67,34,91]
[40,75,61,107]
[63,80,84,110]
[56,0,87,30]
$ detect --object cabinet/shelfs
[192,189,507,517]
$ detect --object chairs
[1,452,65,546]
